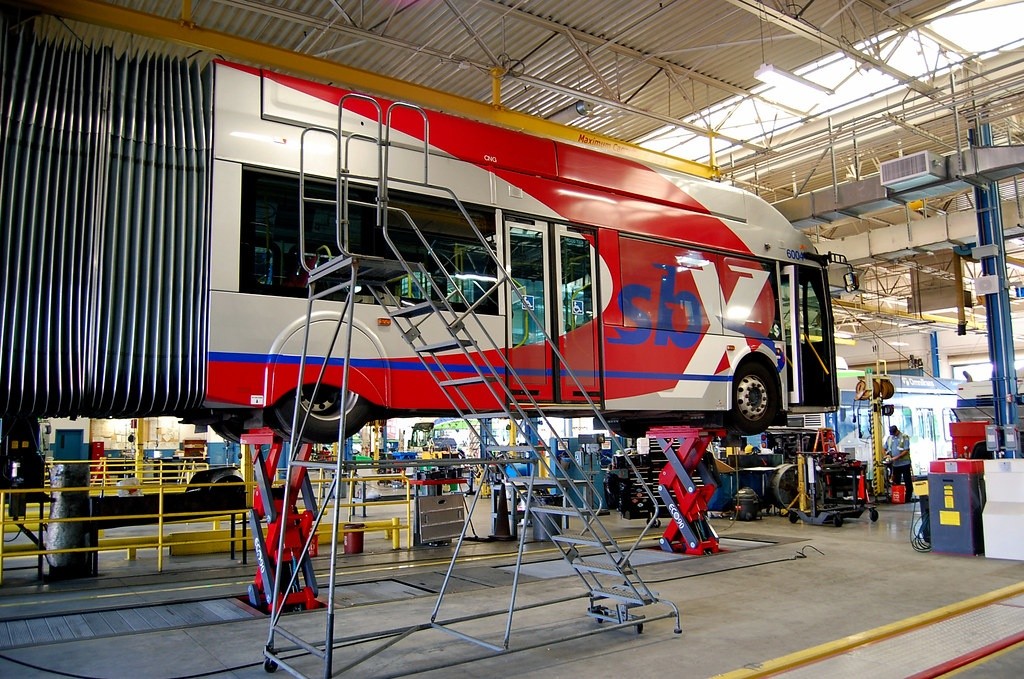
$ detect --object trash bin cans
[530,494,563,542]
[344,524,365,555]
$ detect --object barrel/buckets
[533,495,563,540]
[344,524,364,553]
[308,528,318,556]
[892,485,906,504]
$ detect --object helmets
[745,445,753,453]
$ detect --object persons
[881,424,915,504]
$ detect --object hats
[890,426,897,435]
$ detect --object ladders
[813,428,838,452]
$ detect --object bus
[0,26,860,445]
